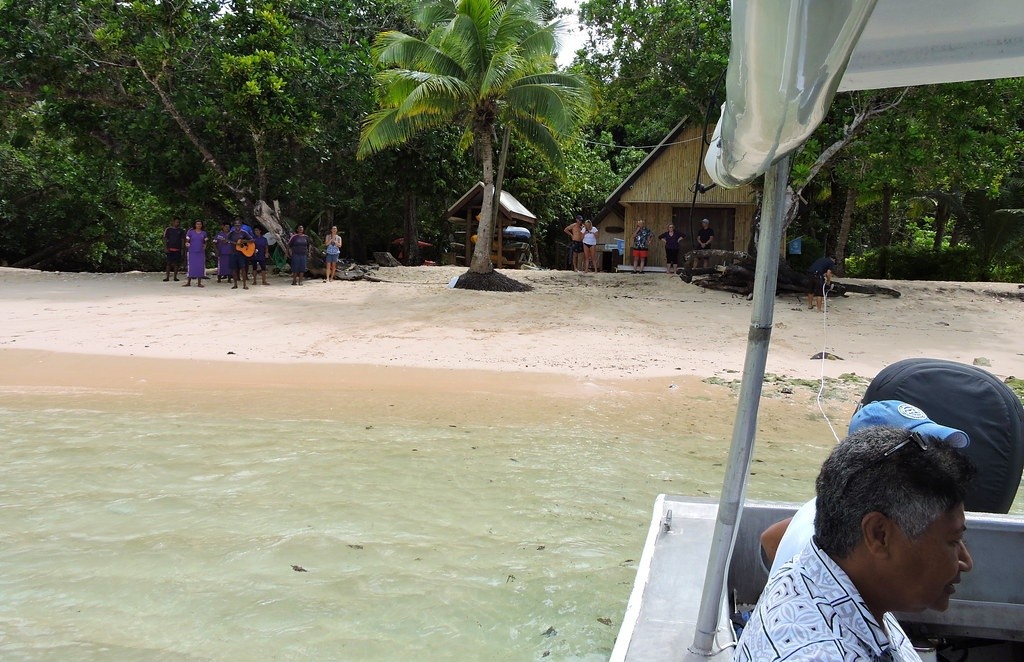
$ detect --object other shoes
[631,270,645,274]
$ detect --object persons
[163,216,280,290]
[284,224,310,286]
[323,225,342,284]
[564,215,600,274]
[806,255,837,309]
[630,218,715,275]
[732,399,973,662]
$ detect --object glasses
[669,227,674,228]
[845,431,930,488]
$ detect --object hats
[830,255,837,265]
[700,219,709,224]
[576,215,583,220]
[234,221,241,226]
[849,400,970,449]
